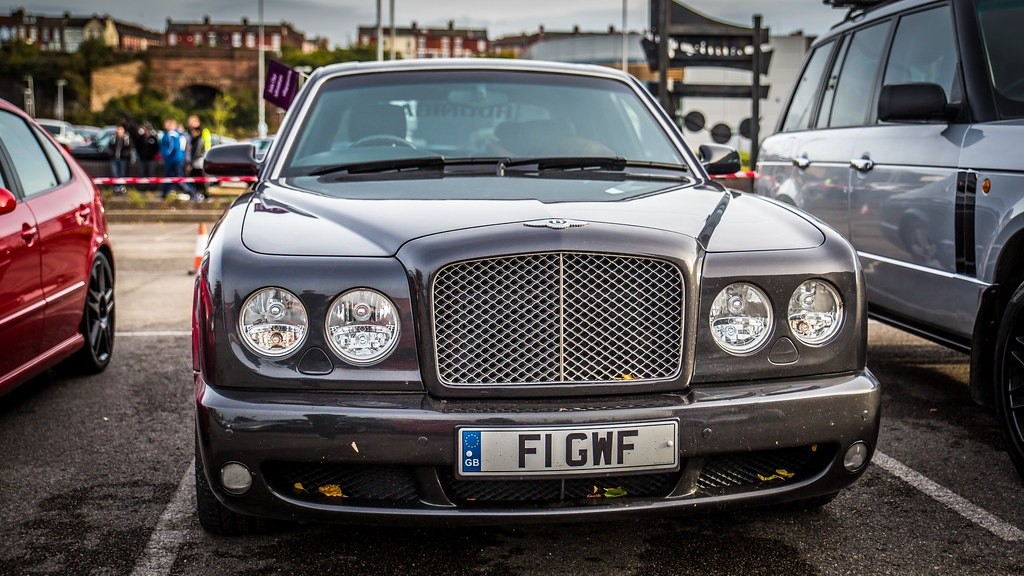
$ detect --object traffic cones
[189,222,210,278]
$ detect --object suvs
[753,0,1024,475]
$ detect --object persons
[108,115,213,203]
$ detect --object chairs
[347,104,407,146]
[494,118,571,156]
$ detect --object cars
[33,113,282,181]
[0,97,117,412]
[188,54,886,538]
